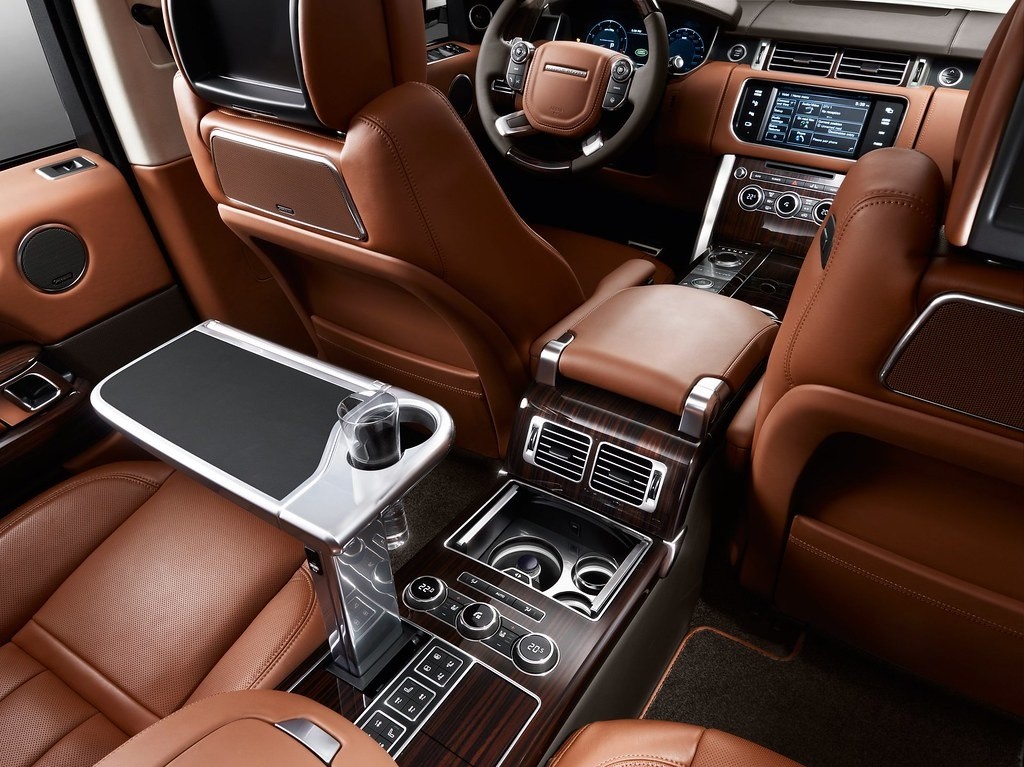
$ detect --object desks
[90,315,457,678]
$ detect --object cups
[337,389,401,471]
[381,499,410,550]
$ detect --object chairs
[161,0,675,461]
[724,0,1024,721]
[0,462,329,767]
